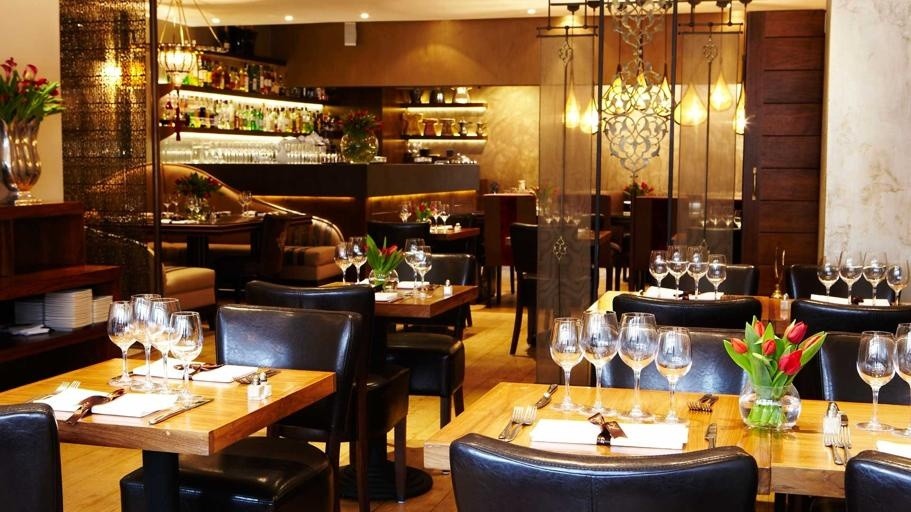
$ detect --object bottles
[465,121,476,136]
[416,113,425,135]
[432,118,443,136]
[158,91,336,134]
[183,54,328,100]
[450,119,460,136]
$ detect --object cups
[162,137,342,163]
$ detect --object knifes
[705,423,716,450]
[174,364,223,371]
[83,388,123,418]
[534,384,558,409]
[147,398,213,425]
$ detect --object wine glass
[816,257,839,297]
[404,239,426,297]
[107,300,140,387]
[615,312,658,422]
[548,317,585,411]
[163,191,204,218]
[885,260,910,307]
[412,246,432,299]
[855,331,897,433]
[334,242,352,285]
[347,236,370,285]
[579,309,618,418]
[838,248,863,302]
[129,294,162,393]
[666,245,687,299]
[697,204,741,230]
[686,246,709,300]
[148,297,181,394]
[706,254,727,300]
[399,200,451,230]
[238,195,245,215]
[863,251,888,305]
[240,191,251,216]
[894,324,911,437]
[653,325,693,427]
[168,311,204,398]
[648,251,668,294]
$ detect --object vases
[0,119,45,207]
[340,134,380,163]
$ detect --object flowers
[342,106,385,137]
[0,58,66,120]
[175,174,223,199]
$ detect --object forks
[507,405,538,442]
[498,406,523,440]
[232,370,271,384]
[835,425,852,464]
[698,396,719,413]
[245,371,280,384]
[823,417,843,466]
[686,393,711,412]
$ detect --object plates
[14,288,112,332]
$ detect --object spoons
[64,396,108,427]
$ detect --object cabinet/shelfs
[402,102,487,142]
[157,56,332,140]
[0,202,124,387]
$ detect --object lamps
[163,2,200,140]
[538,0,746,134]
